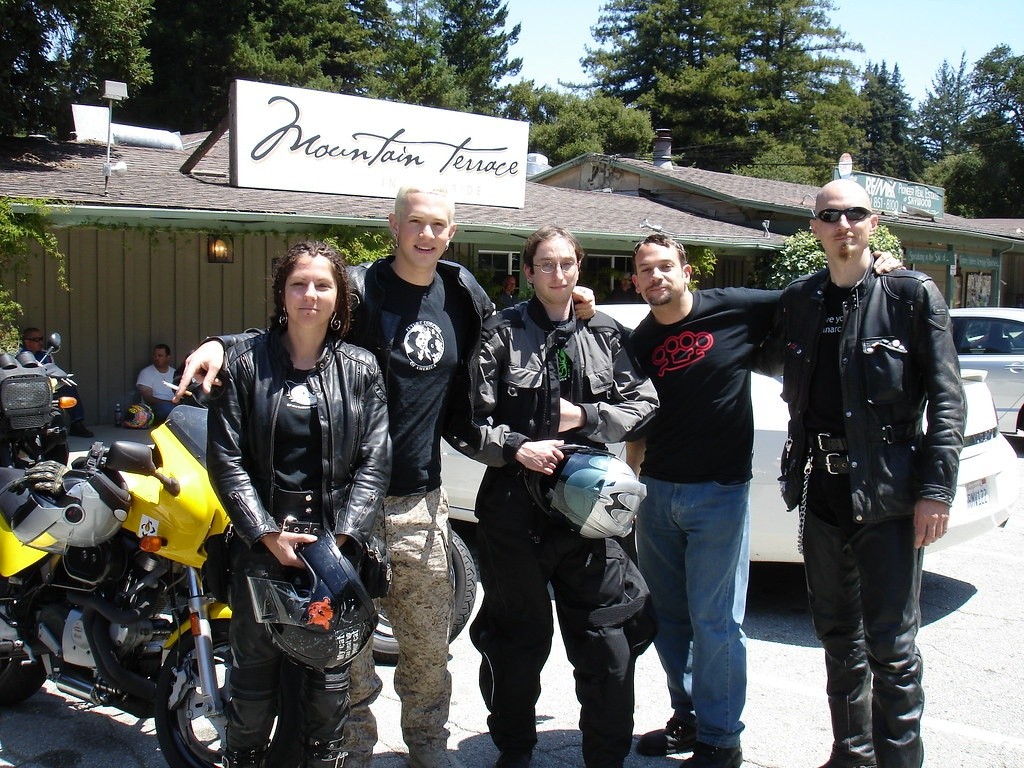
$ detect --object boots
[309,738,347,768]
[222,745,262,768]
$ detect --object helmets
[10,457,134,549]
[521,446,647,539]
[263,529,377,671]
[122,400,154,429]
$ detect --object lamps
[207,234,235,264]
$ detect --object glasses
[24,337,43,342]
[530,261,579,273]
[814,207,873,222]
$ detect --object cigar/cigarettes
[162,381,192,396]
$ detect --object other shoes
[68,425,93,437]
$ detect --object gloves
[24,460,69,492]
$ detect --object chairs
[959,336,972,354]
[984,336,1012,353]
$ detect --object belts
[815,434,851,453]
[817,453,868,474]
[277,521,322,534]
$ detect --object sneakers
[409,742,466,768]
[637,715,701,757]
[679,744,742,768]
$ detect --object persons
[751,179,968,768]
[173,179,596,768]
[603,271,636,303]
[21,328,94,438]
[493,274,521,312]
[135,344,177,425]
[626,234,903,768]
[441,227,660,768]
[205,242,392,768]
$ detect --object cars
[440,304,1023,563]
[947,308,1024,434]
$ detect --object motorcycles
[0,404,310,768]
[0,332,78,470]
